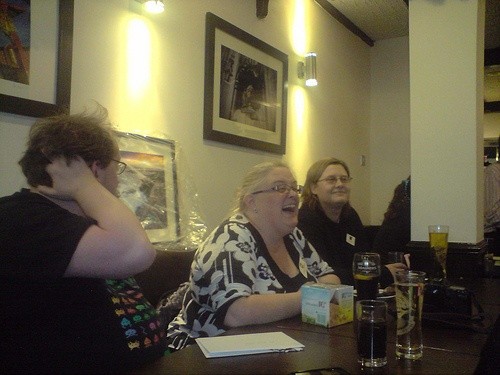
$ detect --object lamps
[296,52,319,88]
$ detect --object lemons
[353,275,373,280]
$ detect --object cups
[395,270,425,364]
[429,224,450,287]
[352,252,380,306]
[355,299,389,367]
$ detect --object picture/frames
[111,125,184,249]
[200,11,289,157]
[0,0,76,120]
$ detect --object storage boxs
[300,282,354,326]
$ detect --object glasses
[316,174,353,183]
[251,182,307,195]
[109,158,127,176]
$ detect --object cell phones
[292,366,351,375]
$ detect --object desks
[134,275,500,375]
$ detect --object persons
[370,180,411,252]
[0,115,169,375]
[297,158,407,286]
[167,163,341,351]
[485,137,500,258]
